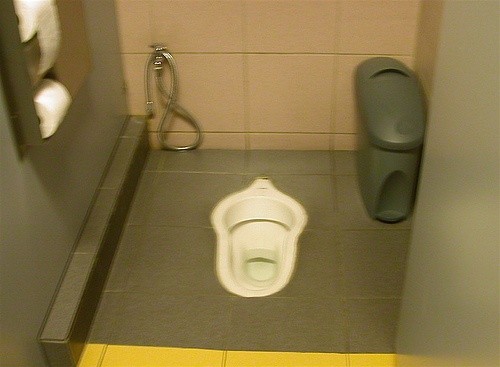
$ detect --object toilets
[208,173,310,299]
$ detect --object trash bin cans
[353,58,427,222]
